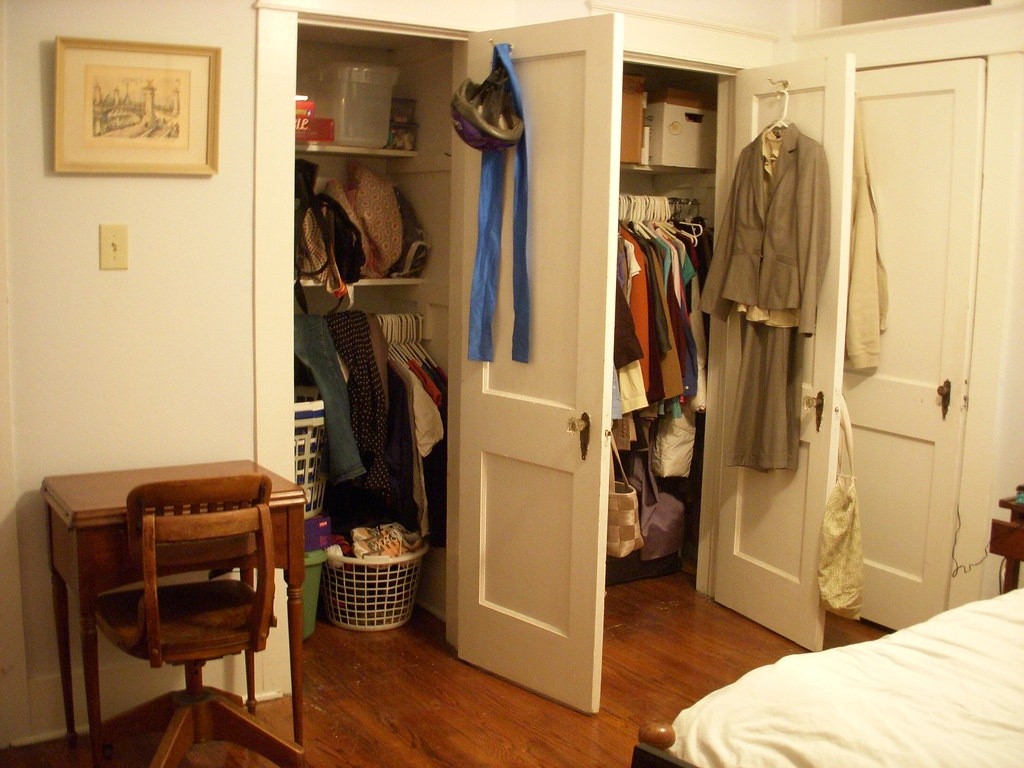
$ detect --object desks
[39,459,305,768]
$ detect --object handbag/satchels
[607,431,644,558]
[817,395,864,621]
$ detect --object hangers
[769,88,791,132]
[374,312,440,372]
[618,194,705,248]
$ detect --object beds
[630,582,1024,768]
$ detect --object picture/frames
[54,35,222,177]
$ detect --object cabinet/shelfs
[294,142,427,288]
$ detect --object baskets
[320,541,429,631]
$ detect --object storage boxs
[621,74,648,164]
[295,101,316,115]
[298,62,399,149]
[649,85,717,111]
[296,115,335,143]
[642,102,717,169]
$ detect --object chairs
[95,473,307,768]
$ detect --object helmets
[451,78,523,152]
